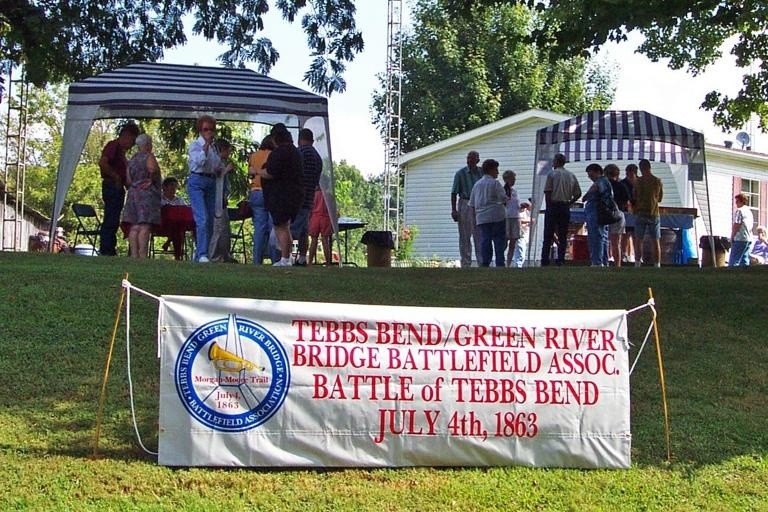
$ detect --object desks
[314,222,368,267]
[119,203,252,261]
[539,206,698,264]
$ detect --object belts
[459,196,471,201]
[191,170,216,178]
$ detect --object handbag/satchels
[597,196,624,227]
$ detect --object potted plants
[430,255,441,267]
[445,259,455,267]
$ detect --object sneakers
[199,255,209,263]
[294,256,306,267]
[272,257,289,267]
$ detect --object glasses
[201,128,217,131]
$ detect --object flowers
[398,225,419,260]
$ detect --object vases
[396,261,414,267]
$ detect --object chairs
[72,203,101,257]
[149,233,187,261]
[228,204,253,264]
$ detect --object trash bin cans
[75,244,99,256]
[367,231,393,267]
[700,236,727,268]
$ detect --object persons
[728,193,754,266]
[749,226,768,266]
[99,115,337,268]
[451,151,663,268]
